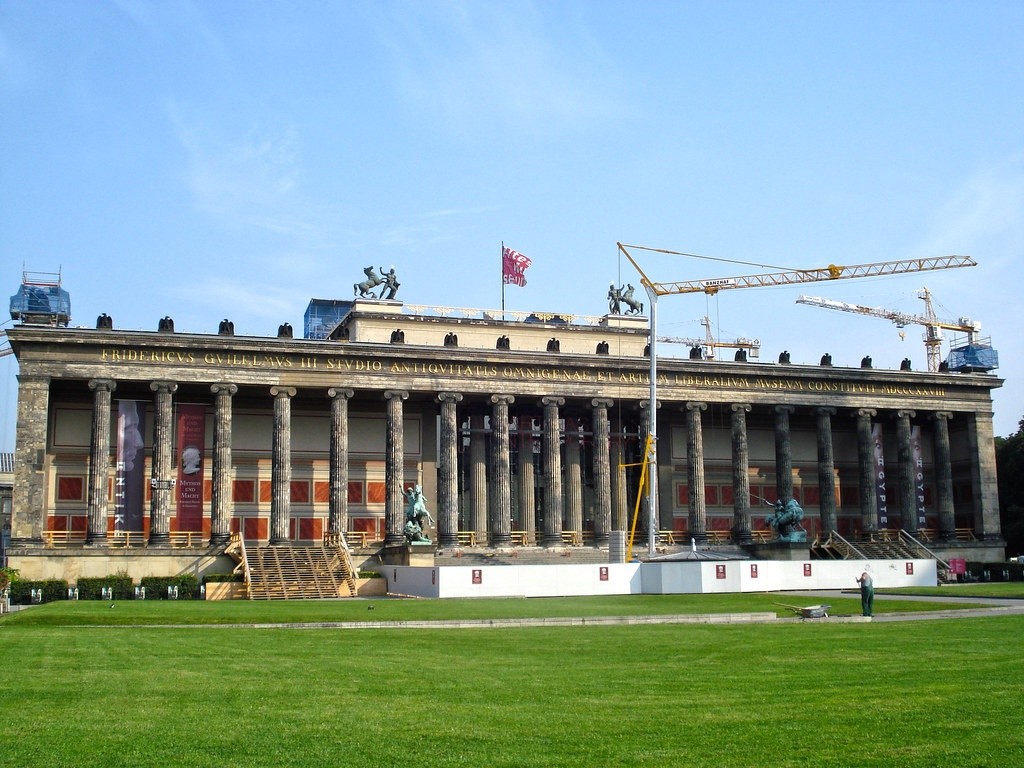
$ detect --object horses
[406,485,435,526]
[617,283,644,317]
[354,266,389,298]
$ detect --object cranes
[796,284,982,373]
[657,316,761,362]
[616,241,980,556]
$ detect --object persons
[379,267,398,299]
[857,572,874,616]
[399,483,416,519]
[608,284,624,315]
[766,500,784,527]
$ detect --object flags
[502,246,532,287]
